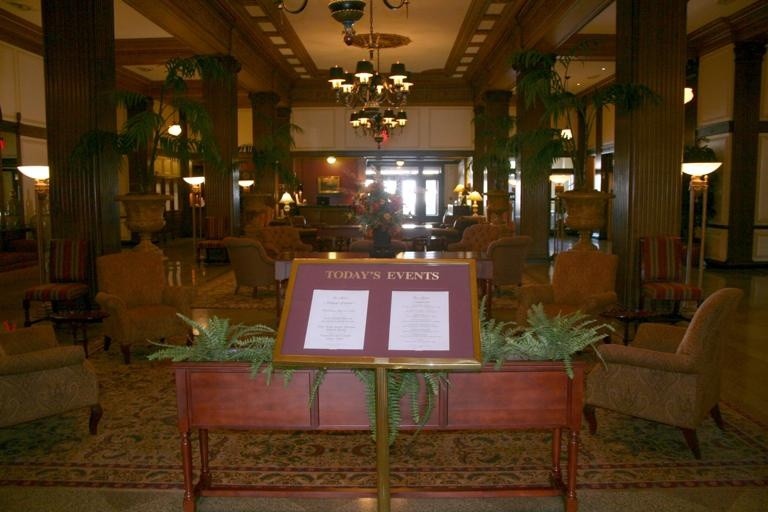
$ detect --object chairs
[261,226,313,254]
[586,289,746,461]
[96,249,193,365]
[224,235,275,298]
[197,215,231,261]
[0,325,104,435]
[516,250,619,328]
[458,223,498,254]
[639,236,703,313]
[487,236,548,319]
[23,239,94,326]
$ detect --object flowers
[345,182,403,236]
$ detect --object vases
[370,229,399,259]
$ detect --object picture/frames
[318,175,341,194]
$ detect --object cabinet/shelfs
[299,205,352,224]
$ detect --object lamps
[16,165,50,318]
[680,161,723,320]
[276,0,412,47]
[158,125,181,136]
[238,179,255,193]
[453,184,465,206]
[279,191,294,218]
[327,34,414,149]
[182,176,205,261]
[467,191,483,216]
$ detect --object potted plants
[62,53,242,255]
[468,113,520,224]
[490,40,663,254]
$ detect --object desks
[599,310,672,347]
[455,216,485,235]
[278,215,307,227]
[300,225,362,252]
[274,251,494,328]
